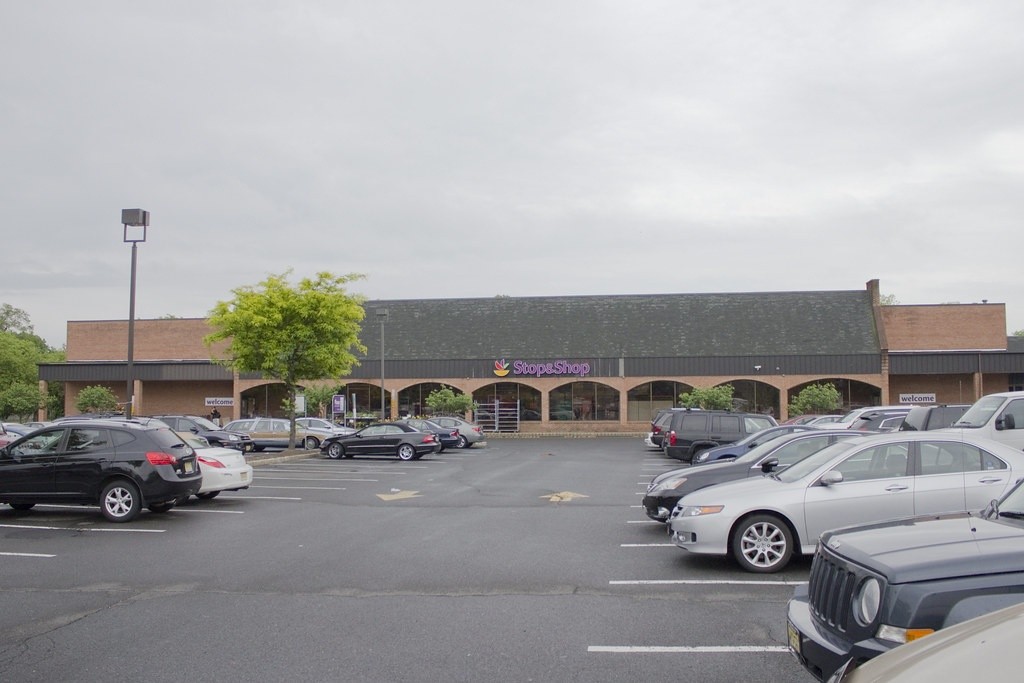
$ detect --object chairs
[885,453,907,476]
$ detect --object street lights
[119,207,152,420]
[375,308,391,423]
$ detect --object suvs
[147,412,255,455]
[663,406,783,465]
[0,413,203,524]
[782,473,1024,683]
[649,404,707,449]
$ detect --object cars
[0,412,125,448]
[318,421,442,461]
[372,418,462,454]
[689,390,1024,464]
[663,429,1024,574]
[414,416,486,449]
[641,427,909,524]
[825,602,1023,683]
[176,429,252,500]
[283,416,358,437]
[222,418,336,453]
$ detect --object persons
[768,407,774,416]
[211,407,220,427]
